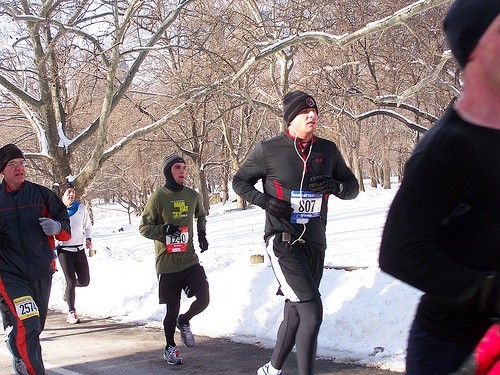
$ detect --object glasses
[8,160,27,168]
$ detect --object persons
[139,156,210,363]
[0,144,71,375]
[53,182,94,324]
[378,0,500,375]
[232,90,358,375]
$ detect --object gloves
[198,236,209,253]
[259,194,294,218]
[39,218,61,236]
[308,175,343,195]
[163,224,181,238]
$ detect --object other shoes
[257,360,283,375]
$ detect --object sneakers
[176,314,195,347]
[163,344,184,365]
[66,310,80,324]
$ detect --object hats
[163,155,185,173]
[283,90,318,126]
[0,143,25,173]
[443,0,500,69]
[59,182,74,196]
